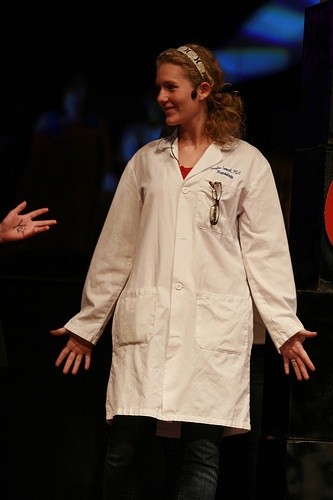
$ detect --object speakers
[262,291,333,442]
[255,436,333,500]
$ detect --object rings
[291,359,296,362]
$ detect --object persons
[0,201,57,243]
[50,44,318,500]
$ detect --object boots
[141,432,186,500]
[214,432,250,500]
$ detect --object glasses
[209,181,222,227]
